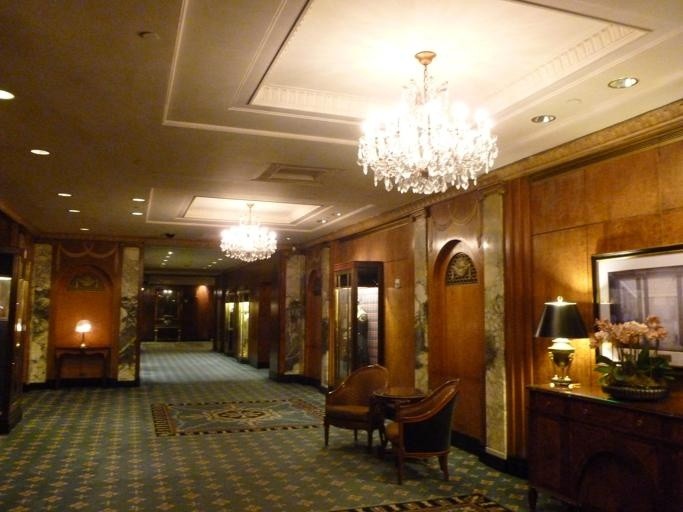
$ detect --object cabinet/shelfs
[521,385,682,512]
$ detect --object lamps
[530,293,588,390]
[354,49,498,201]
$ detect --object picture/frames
[589,245,683,378]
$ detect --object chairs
[323,364,461,485]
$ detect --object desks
[53,345,110,387]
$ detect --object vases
[601,372,670,402]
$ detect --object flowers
[587,315,677,384]
